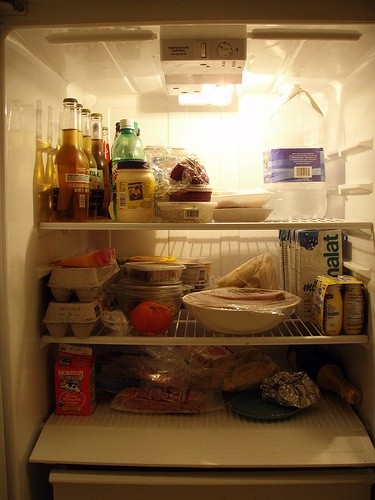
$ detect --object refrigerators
[0,1,375,500]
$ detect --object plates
[222,389,302,423]
[156,184,274,223]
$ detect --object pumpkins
[130,301,172,333]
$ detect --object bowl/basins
[108,256,213,317]
[181,288,301,335]
[270,181,327,220]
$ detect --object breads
[169,159,210,184]
[94,351,232,411]
[213,259,278,291]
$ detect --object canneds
[115,159,155,223]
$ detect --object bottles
[55,98,155,223]
[286,344,361,406]
[316,275,366,335]
